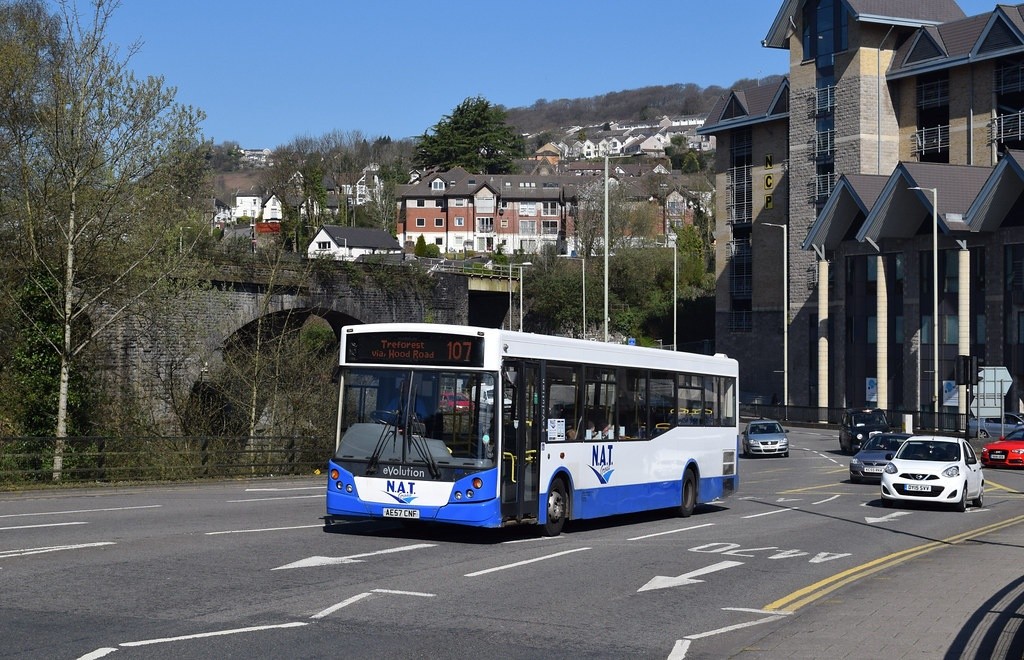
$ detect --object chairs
[448,407,716,441]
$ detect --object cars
[850,433,925,482]
[741,419,790,457]
[880,435,985,512]
[980,426,1024,469]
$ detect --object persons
[382,381,429,425]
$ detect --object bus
[322,323,742,538]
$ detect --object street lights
[509,261,533,331]
[908,186,940,433]
[604,149,648,343]
[763,221,789,427]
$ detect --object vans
[838,408,896,455]
[967,411,1024,439]
